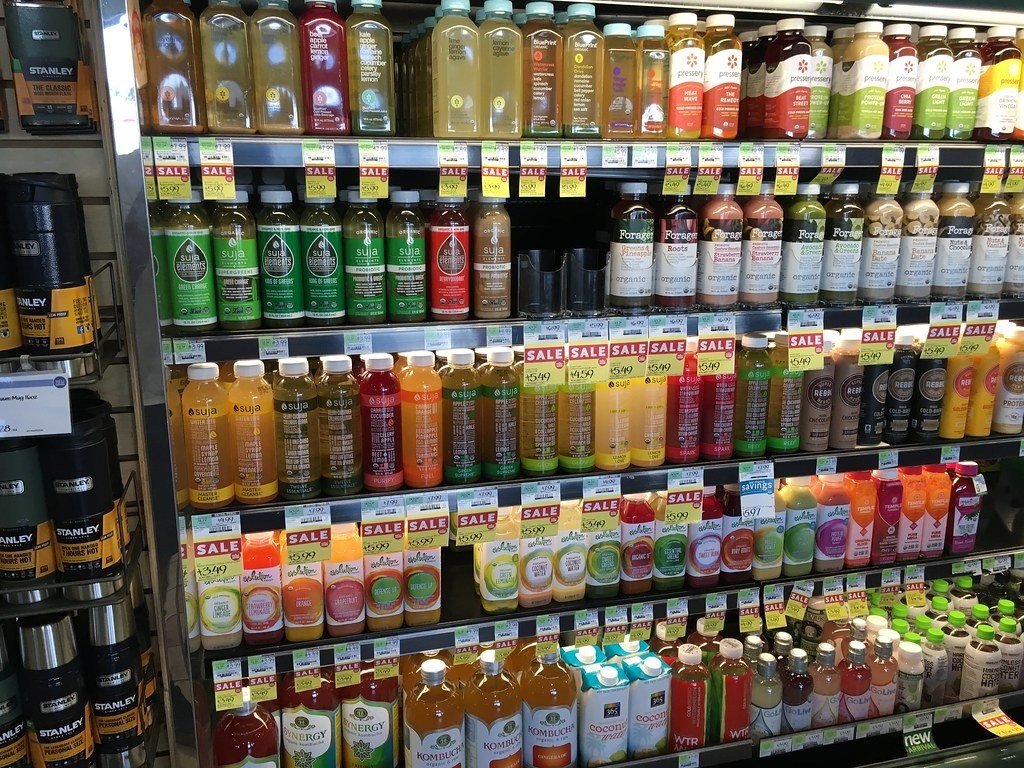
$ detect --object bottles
[142,0,1024,140]
[147,185,1024,768]
